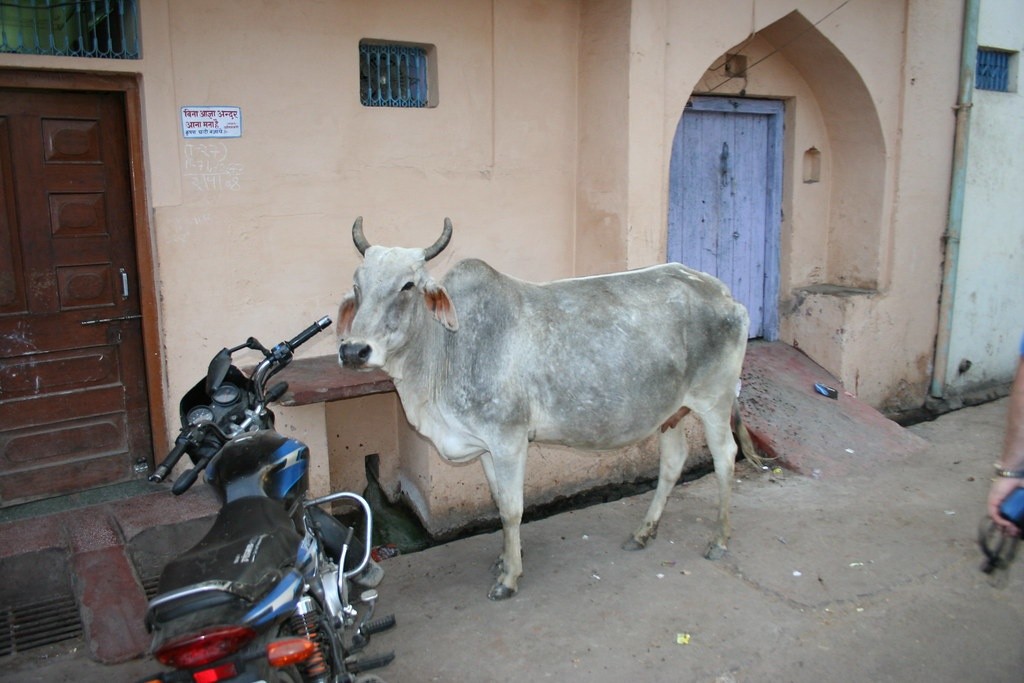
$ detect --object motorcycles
[133,312,401,683]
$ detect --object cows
[334,216,779,602]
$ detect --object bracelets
[995,467,1023,478]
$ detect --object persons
[986,338,1024,534]
